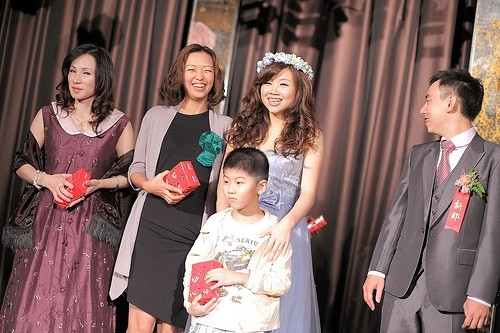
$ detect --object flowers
[257,52,314,80]
[453,167,486,199]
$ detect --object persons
[0,44,136,333]
[214,62,324,333]
[363,66,500,333]
[125,43,324,333]
[182,148,293,333]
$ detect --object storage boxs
[188,260,223,303]
[53,168,91,208]
[163,161,200,205]
[308,214,326,232]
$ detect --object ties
[435,140,456,186]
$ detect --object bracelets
[32,169,45,190]
[109,176,121,192]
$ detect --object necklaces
[73,109,96,126]
